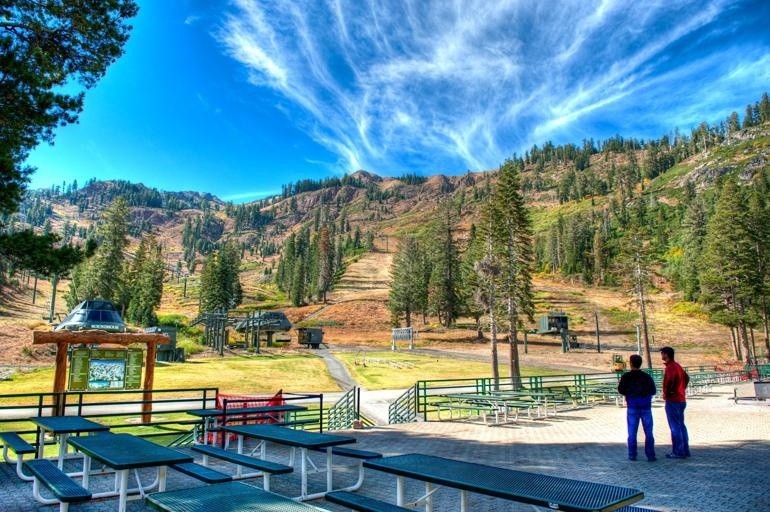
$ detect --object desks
[359,452,644,512]
[144,481,333,512]
[187,405,308,449]
[65,433,194,512]
[220,423,356,498]
[28,415,110,471]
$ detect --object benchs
[613,505,662,512]
[0,432,36,483]
[326,490,417,512]
[309,446,382,492]
[176,414,283,441]
[430,369,740,424]
[169,462,232,485]
[189,445,293,491]
[24,459,92,512]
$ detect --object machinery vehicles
[612,354,627,373]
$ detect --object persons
[660,346,692,460]
[617,354,660,461]
